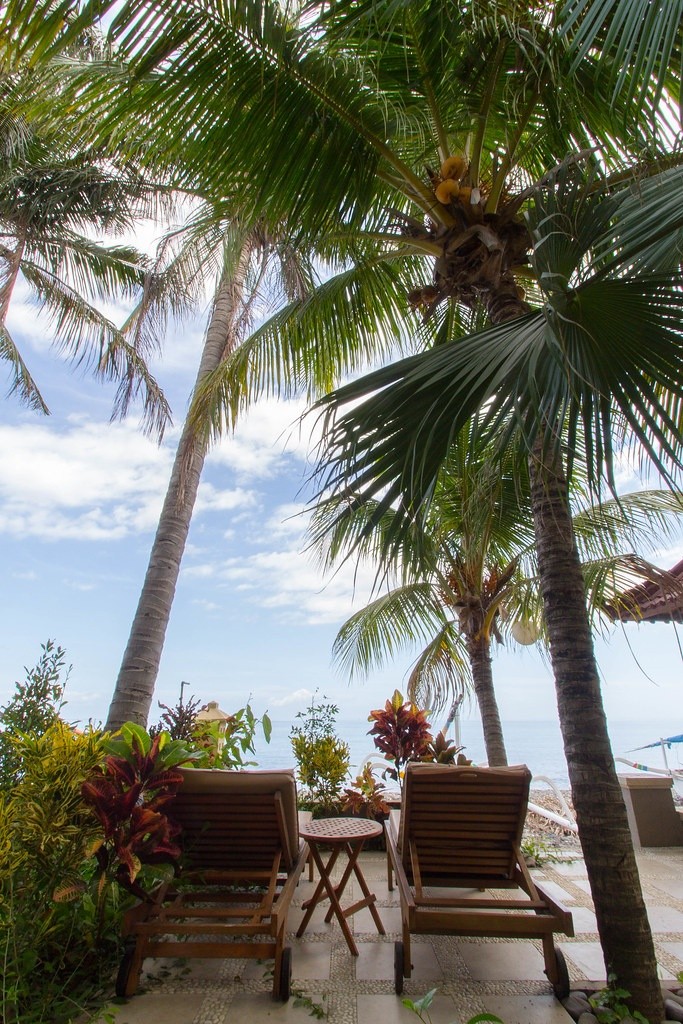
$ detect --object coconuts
[511,621,540,645]
[406,156,525,305]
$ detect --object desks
[295,816,388,957]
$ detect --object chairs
[114,767,317,1003]
[385,763,575,999]
